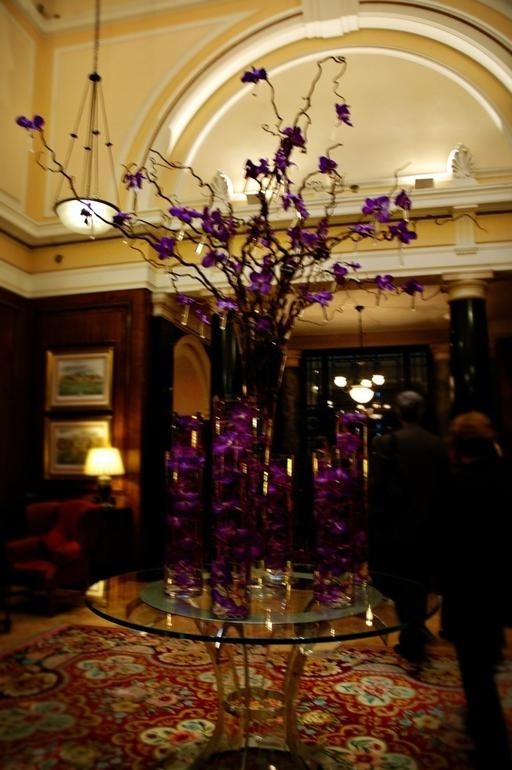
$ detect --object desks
[84,571,442,769]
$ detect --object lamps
[84,448,125,503]
[334,306,385,404]
[52,0,121,238]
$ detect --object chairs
[8,502,112,619]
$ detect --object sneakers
[394,626,435,663]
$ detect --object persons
[422,412,512,770]
[363,389,446,673]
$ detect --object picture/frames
[45,346,113,411]
[44,416,114,481]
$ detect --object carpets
[1,622,512,769]
